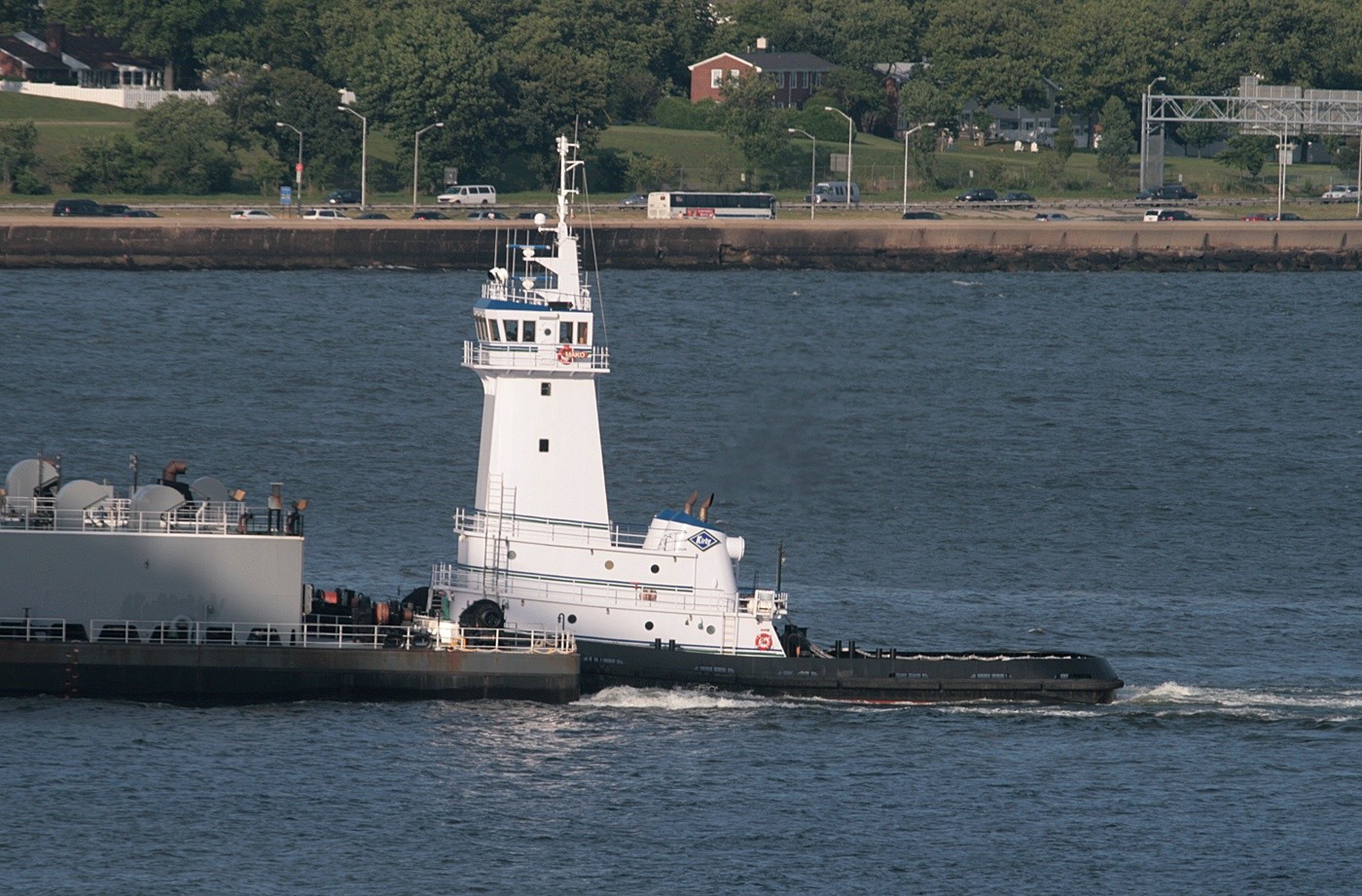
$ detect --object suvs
[300,189,388,220]
[1144,209,1203,222]
[52,199,110,217]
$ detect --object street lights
[903,122,936,214]
[277,123,302,213]
[788,128,814,221]
[825,107,852,210]
[1253,105,1289,221]
[338,106,366,210]
[1147,77,1166,169]
[413,123,444,210]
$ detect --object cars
[412,209,554,221]
[902,211,942,220]
[1035,213,1070,222]
[1322,186,1362,204]
[955,189,1037,209]
[105,204,164,218]
[1240,212,1301,221]
[1133,186,1198,207]
[228,209,275,218]
[619,194,648,210]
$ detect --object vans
[437,185,496,205]
[804,182,860,210]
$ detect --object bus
[648,192,783,219]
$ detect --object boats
[404,114,1124,707]
[0,449,582,704]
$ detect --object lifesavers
[481,609,502,628]
[557,347,573,365]
[755,633,773,649]
